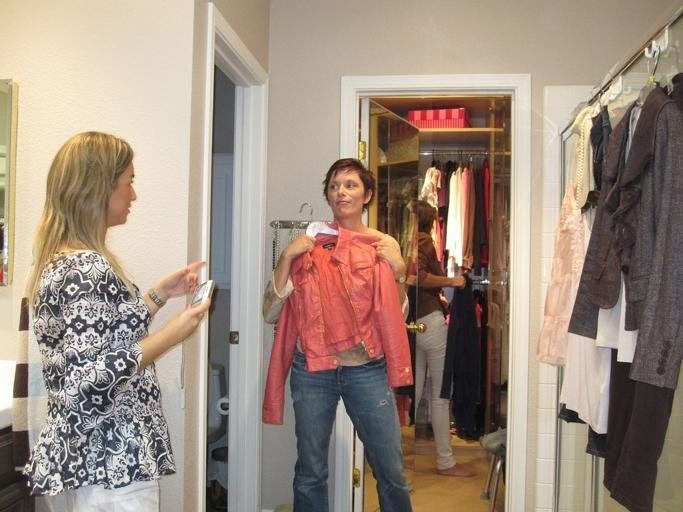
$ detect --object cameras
[191,280,216,309]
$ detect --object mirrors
[364,102,419,512]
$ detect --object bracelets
[148,287,166,309]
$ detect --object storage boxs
[407,107,471,129]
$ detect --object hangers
[632,46,660,108]
[299,202,339,252]
[590,92,604,116]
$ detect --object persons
[22,132,212,512]
[262,157,412,512]
[403,200,480,477]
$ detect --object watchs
[395,274,407,284]
